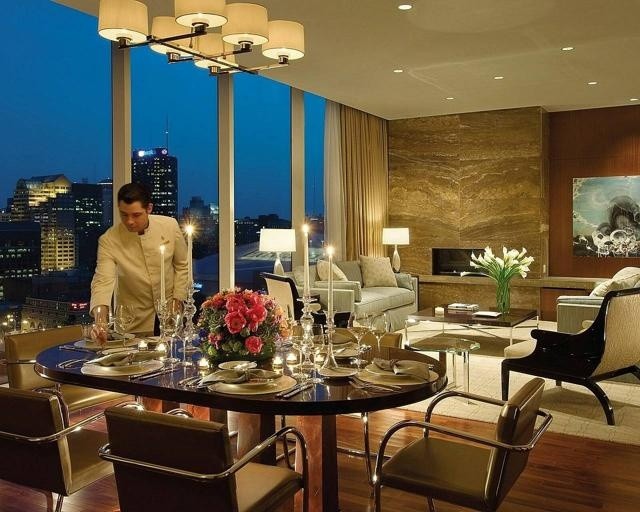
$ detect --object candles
[160,245,166,308]
[325,246,335,320]
[184,225,195,288]
[301,223,310,297]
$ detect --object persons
[89,183,193,414]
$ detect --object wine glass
[81,304,136,356]
[347,312,389,366]
[289,322,325,383]
[156,299,193,366]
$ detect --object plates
[81,359,164,377]
[318,361,439,385]
[74,338,142,349]
[473,310,502,317]
[206,360,297,395]
[333,349,358,359]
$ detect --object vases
[496,284,510,315]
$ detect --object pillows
[313,254,413,303]
[588,273,640,297]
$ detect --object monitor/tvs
[430,247,492,276]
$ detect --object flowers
[460,246,535,310]
[196,286,292,361]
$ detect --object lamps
[382,227,410,274]
[97,0,305,76]
[259,228,296,276]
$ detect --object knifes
[129,365,205,386]
[276,382,313,398]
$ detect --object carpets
[392,318,639,446]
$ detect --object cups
[271,352,297,372]
[435,307,445,318]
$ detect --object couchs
[283,260,419,338]
[556,266,640,383]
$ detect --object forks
[349,377,401,391]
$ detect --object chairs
[259,271,351,329]
[371,377,553,511]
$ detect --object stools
[405,333,481,405]
